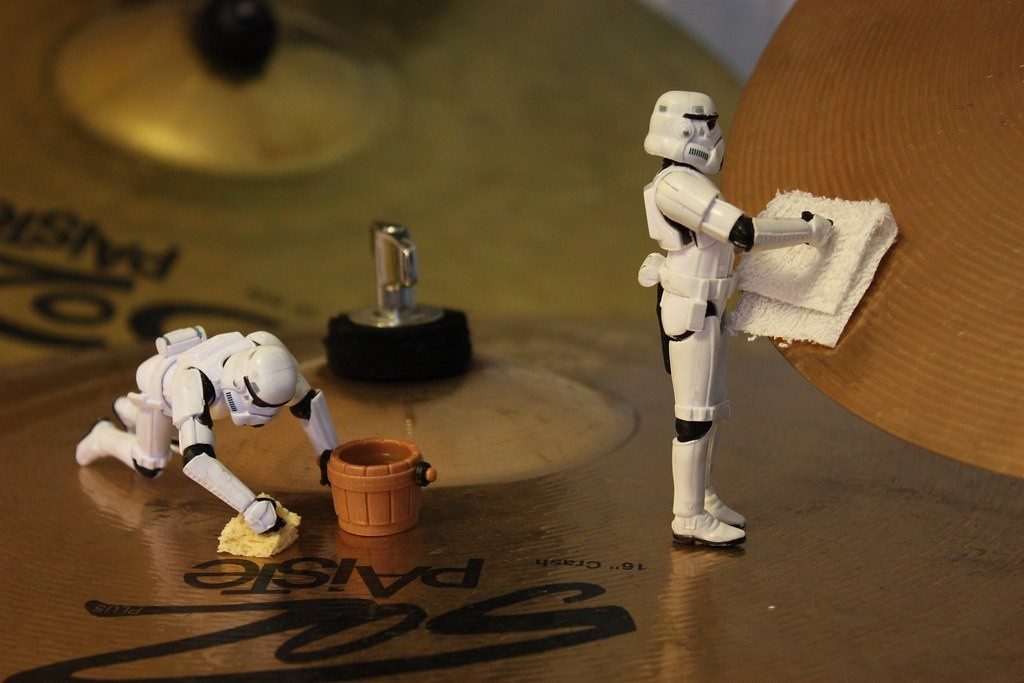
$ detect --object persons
[637,89,837,550]
[74,326,342,536]
